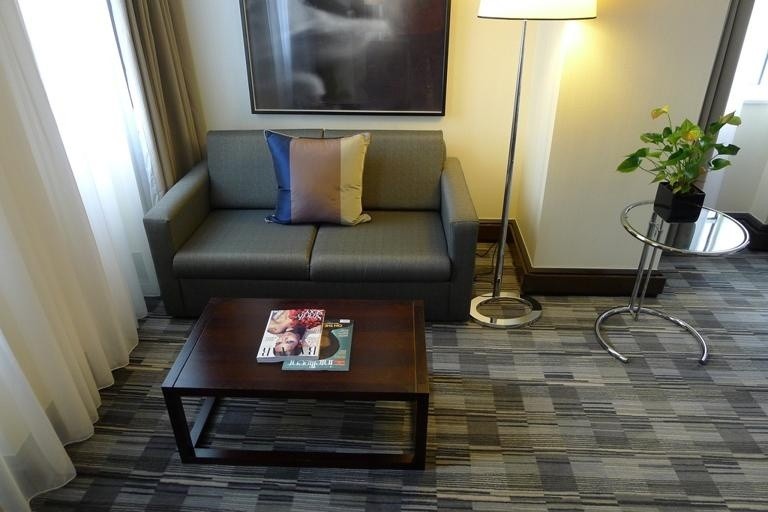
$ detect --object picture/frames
[239,0,451,117]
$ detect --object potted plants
[616,104,742,223]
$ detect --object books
[255,307,326,363]
[281,318,355,373]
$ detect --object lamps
[468,0,598,330]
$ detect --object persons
[268,310,323,356]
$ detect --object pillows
[262,129,373,227]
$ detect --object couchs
[142,130,481,322]
